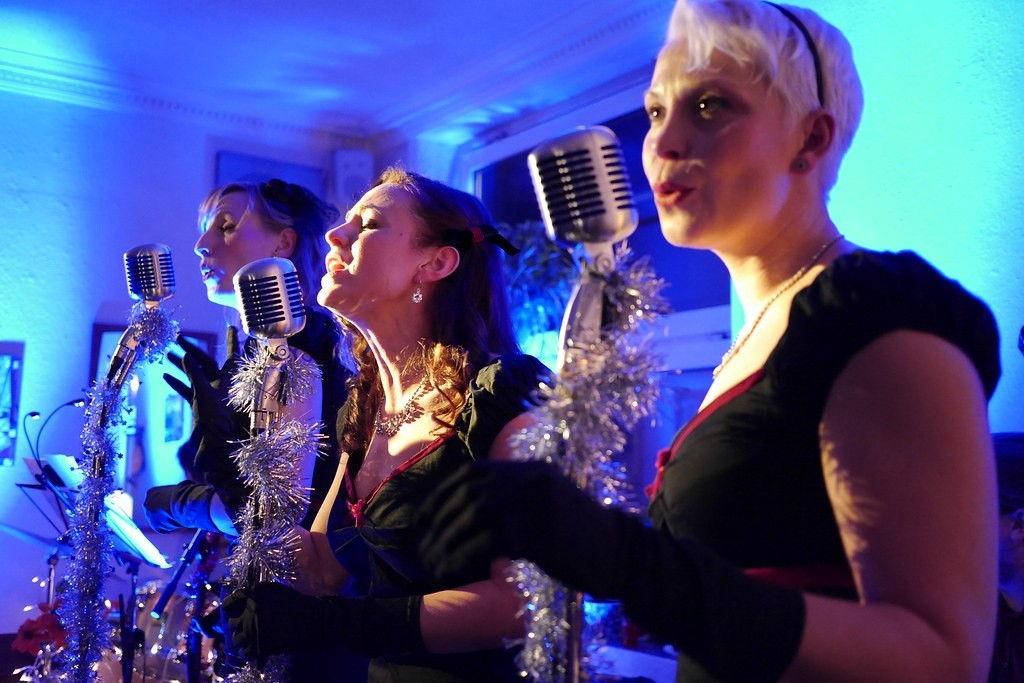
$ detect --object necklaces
[709,234,846,378]
[374,374,439,437]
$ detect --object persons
[145,182,350,537]
[367,1,1003,683]
[197,169,559,682]
[0,598,69,683]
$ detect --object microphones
[233,257,307,338]
[124,244,176,301]
[528,125,639,249]
